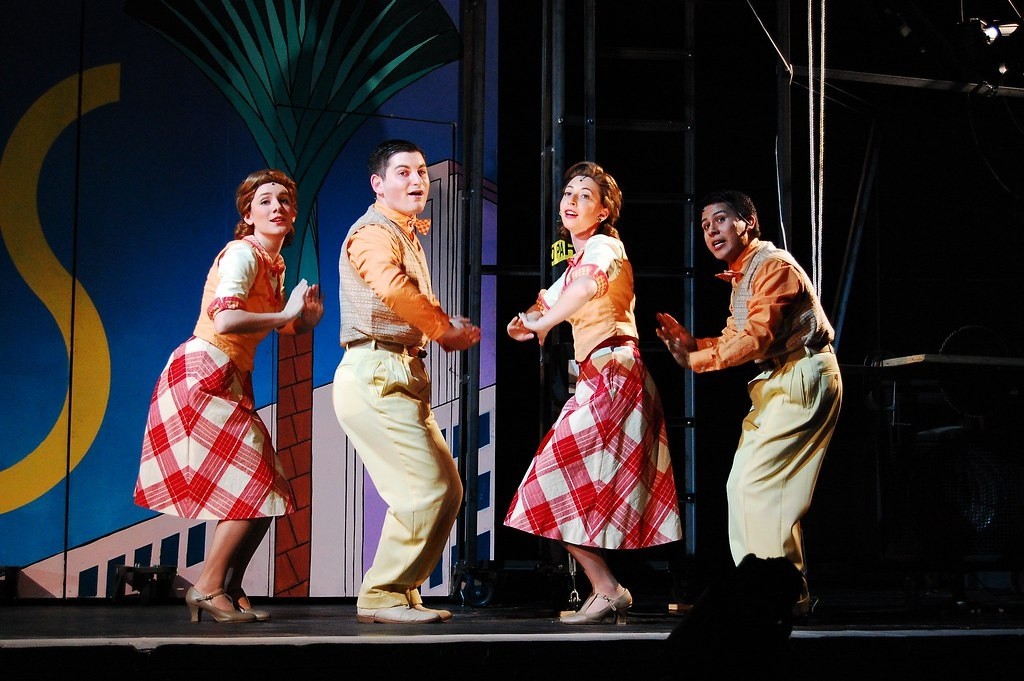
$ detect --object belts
[760,342,835,370]
[346,338,428,358]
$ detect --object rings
[472,339,476,344]
[471,326,475,332]
[469,335,472,339]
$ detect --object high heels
[186,585,256,624]
[560,587,632,625]
[223,582,271,621]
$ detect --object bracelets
[703,338,713,347]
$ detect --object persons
[656,190,844,619]
[333,140,481,623]
[133,170,326,625]
[504,161,680,626]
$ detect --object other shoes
[357,601,439,624]
[412,600,451,621]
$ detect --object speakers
[873,367,1024,564]
